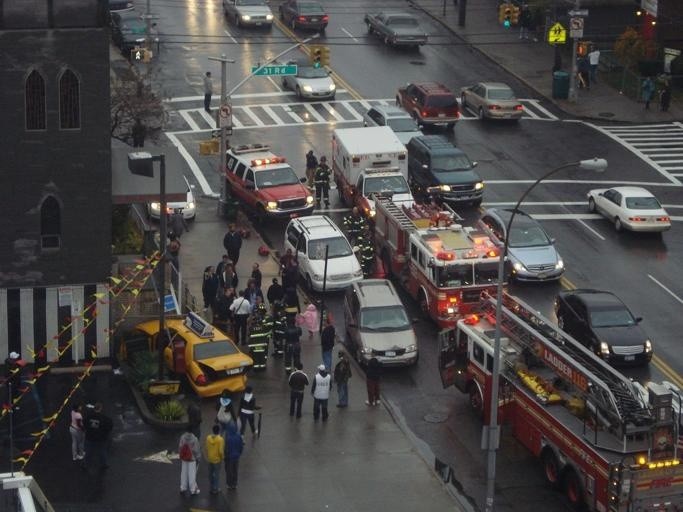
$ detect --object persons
[69,401,88,461]
[287,351,351,421]
[169,206,188,241]
[304,151,319,185]
[344,207,376,278]
[659,78,672,114]
[202,249,318,374]
[205,424,224,494]
[642,74,653,111]
[201,71,212,112]
[176,424,202,495]
[223,222,242,267]
[222,420,245,490]
[365,358,381,405]
[313,155,332,206]
[320,319,334,372]
[215,386,261,435]
[85,400,111,435]
[588,41,600,91]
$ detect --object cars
[554,289,654,367]
[279,1,329,31]
[223,0,274,28]
[587,186,672,233]
[365,9,429,47]
[460,82,523,122]
[146,174,197,224]
[119,311,254,399]
[476,206,565,282]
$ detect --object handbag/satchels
[218,406,232,424]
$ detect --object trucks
[332,125,417,227]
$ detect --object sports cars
[281,58,336,100]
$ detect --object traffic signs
[140,12,160,21]
[212,128,232,140]
[566,9,589,19]
[251,65,298,77]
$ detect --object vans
[284,215,364,293]
[363,105,425,149]
[344,278,420,369]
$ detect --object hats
[317,364,325,371]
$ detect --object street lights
[128,151,165,381]
[477,158,608,512]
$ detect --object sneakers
[377,400,381,405]
[365,400,375,405]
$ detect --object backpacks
[180,444,192,462]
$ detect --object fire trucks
[370,186,514,329]
[437,289,683,512]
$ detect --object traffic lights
[321,45,330,69]
[310,46,322,69]
[504,4,512,28]
[576,42,587,57]
[511,5,520,25]
[499,4,505,24]
[130,48,150,64]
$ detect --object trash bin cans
[553,71,568,99]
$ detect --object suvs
[225,143,314,223]
[407,135,483,207]
[396,81,459,129]
